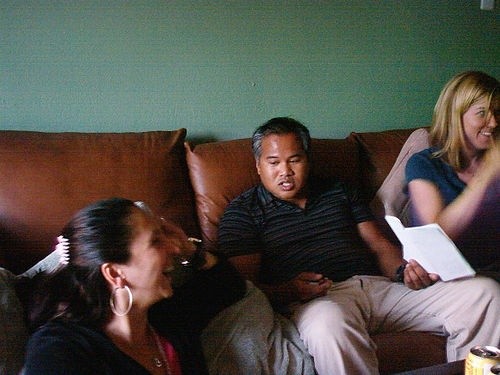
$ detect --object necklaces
[139,346,162,367]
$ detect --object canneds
[464,346,500,375]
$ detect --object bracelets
[179,238,205,268]
[396,265,405,282]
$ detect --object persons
[21,198,248,375]
[403,72,500,283]
[218,117,500,375]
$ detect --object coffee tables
[394,358,465,375]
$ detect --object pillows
[0,128,205,276]
[184,135,359,256]
[350,127,431,200]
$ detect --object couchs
[259,257,448,375]
